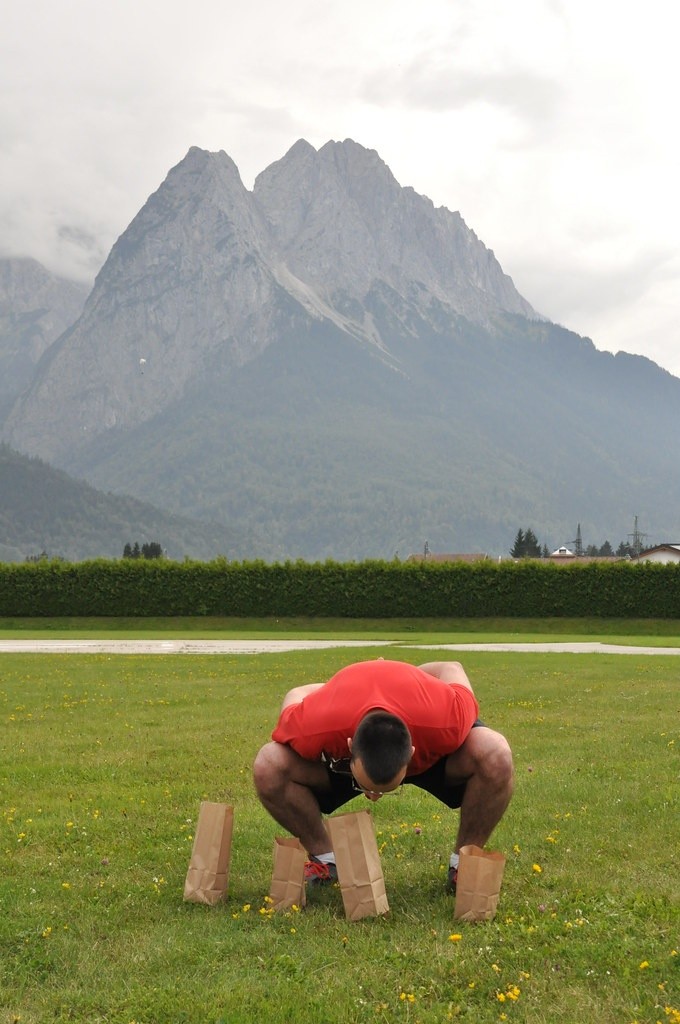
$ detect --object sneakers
[304,855,338,887]
[447,867,458,896]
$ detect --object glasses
[350,777,404,795]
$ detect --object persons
[254,659,514,895]
